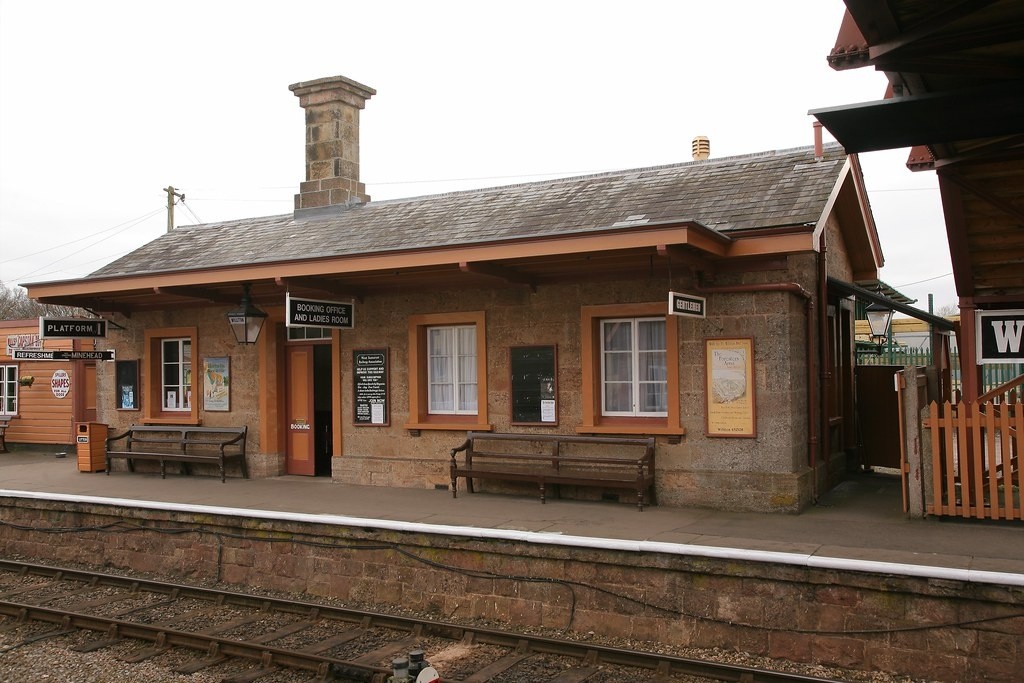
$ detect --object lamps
[853,285,896,356]
[226,283,268,345]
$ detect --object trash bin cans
[74,421,109,472]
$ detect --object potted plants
[17,377,35,388]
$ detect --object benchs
[104,424,248,484]
[450,431,656,511]
[0,416,11,453]
